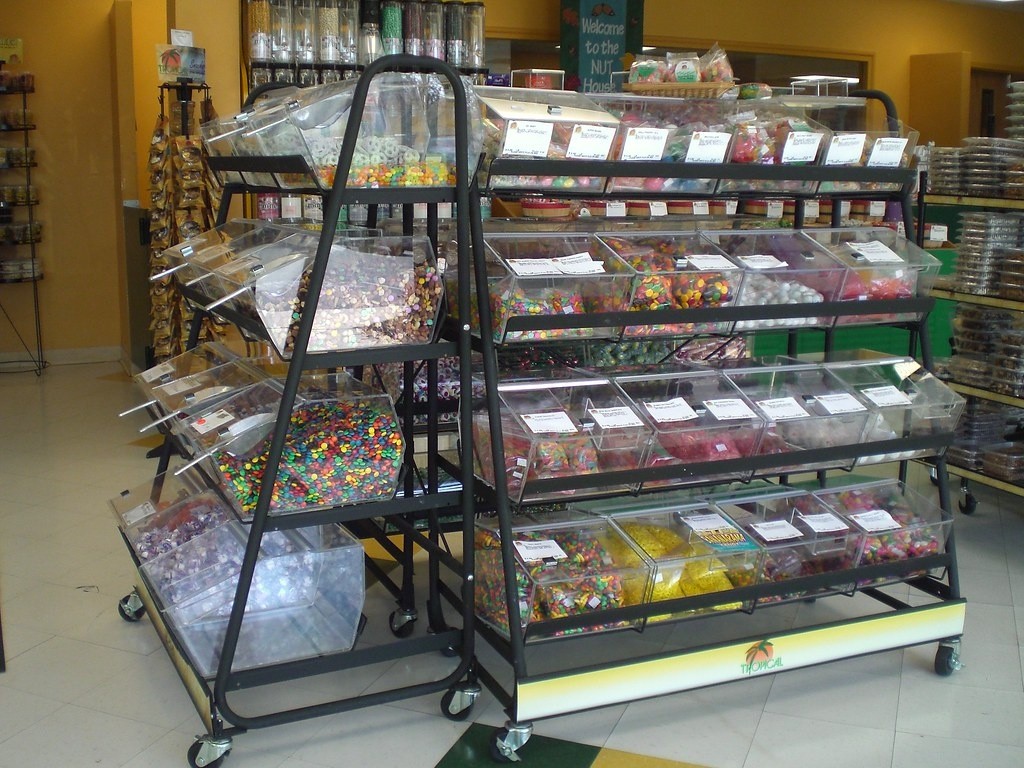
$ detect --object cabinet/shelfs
[116,55,482,768]
[905,191,1024,514]
[423,80,967,762]
[0,85,52,377]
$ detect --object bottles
[255,191,492,238]
[247,0,485,91]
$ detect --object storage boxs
[470,86,923,193]
[442,218,943,345]
[454,366,966,505]
[475,475,952,642]
[163,218,444,361]
[108,450,366,678]
[136,341,407,518]
[198,71,467,186]
